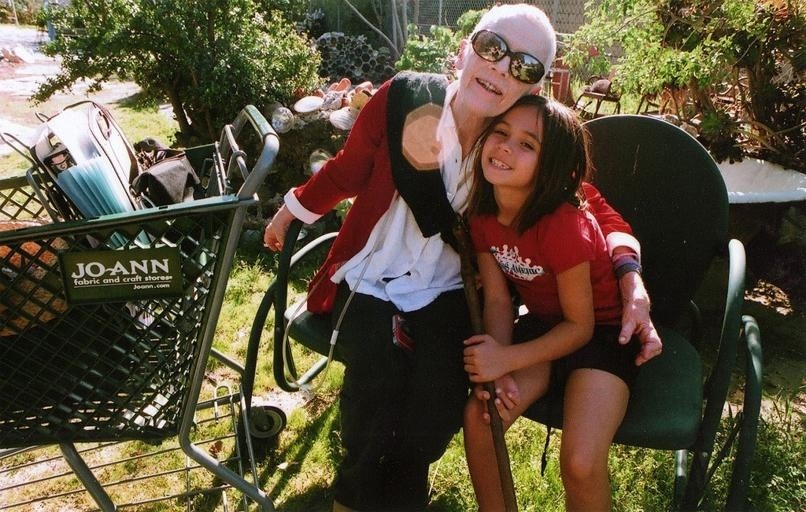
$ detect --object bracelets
[612,251,642,278]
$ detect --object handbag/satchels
[130,138,207,229]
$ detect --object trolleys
[0,99,293,509]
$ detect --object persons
[265,1,665,512]
[459,93,636,511]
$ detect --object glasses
[470,30,544,84]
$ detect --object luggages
[35,100,145,236]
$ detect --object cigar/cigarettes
[262,243,281,247]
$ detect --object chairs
[241,207,516,499]
[568,73,752,131]
[513,114,763,512]
